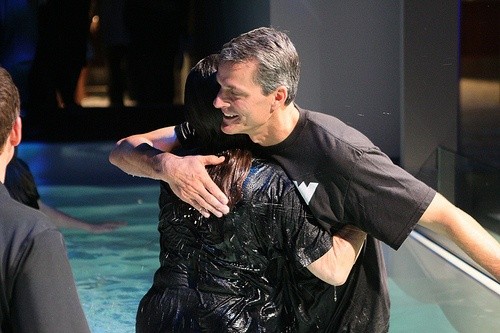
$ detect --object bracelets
[344,225,367,240]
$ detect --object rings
[197,207,204,213]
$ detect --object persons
[4,146,126,234]
[1,65,91,333]
[134,54,367,333]
[108,27,499,332]
